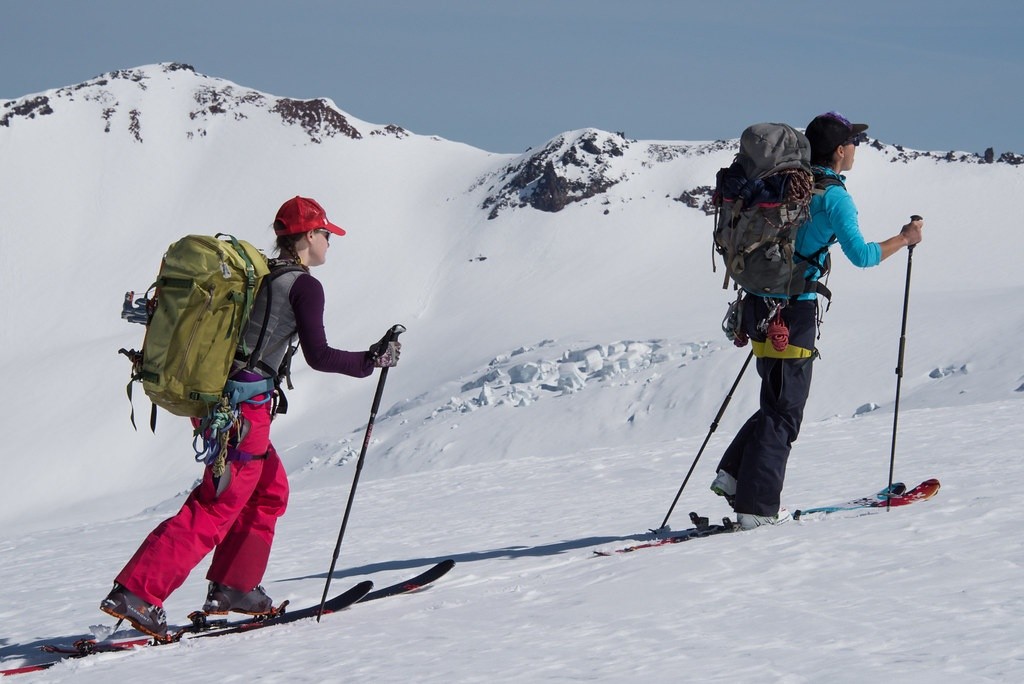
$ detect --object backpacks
[712,124,847,311]
[117,232,310,435]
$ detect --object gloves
[373,342,401,367]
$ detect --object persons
[711,112,924,532]
[99,194,401,641]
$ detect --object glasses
[317,229,331,240]
[842,137,859,146]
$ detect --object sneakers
[99,584,168,640]
[710,469,737,509]
[737,508,793,529]
[203,580,272,615]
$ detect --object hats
[805,112,868,154]
[273,195,346,236]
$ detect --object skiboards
[11,556,459,678]
[592,475,942,560]
